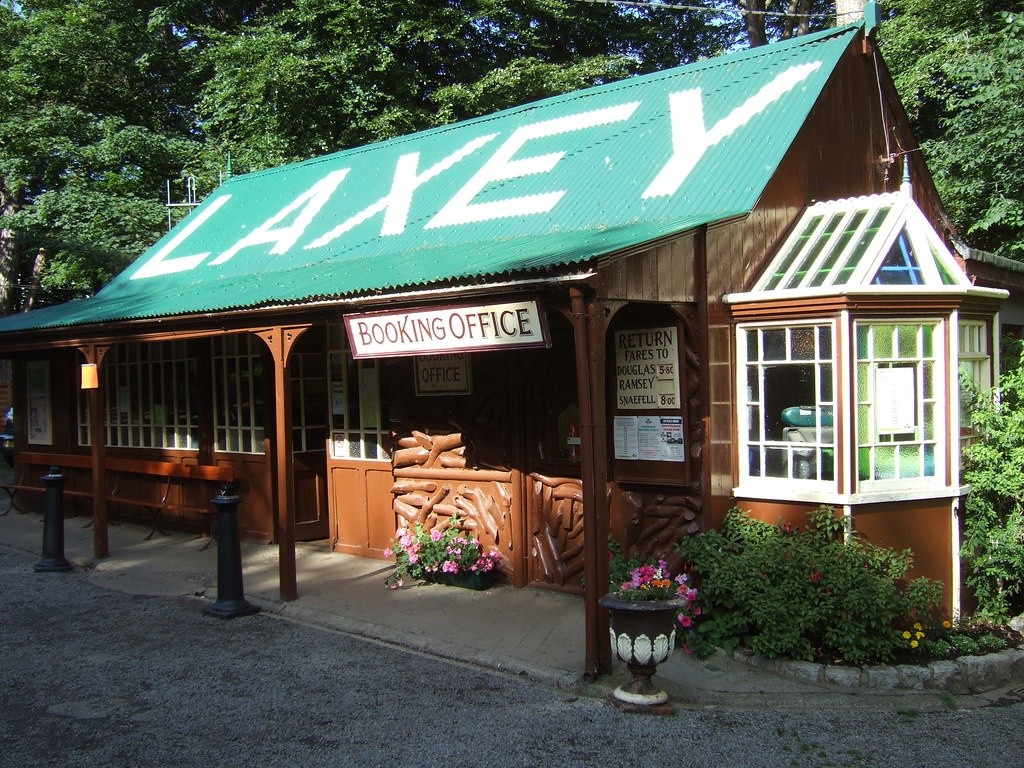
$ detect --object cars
[0,406,14,467]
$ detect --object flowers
[616,561,701,655]
[383,512,499,590]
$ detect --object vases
[596,592,689,714]
[409,566,496,591]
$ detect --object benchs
[0,452,234,552]
